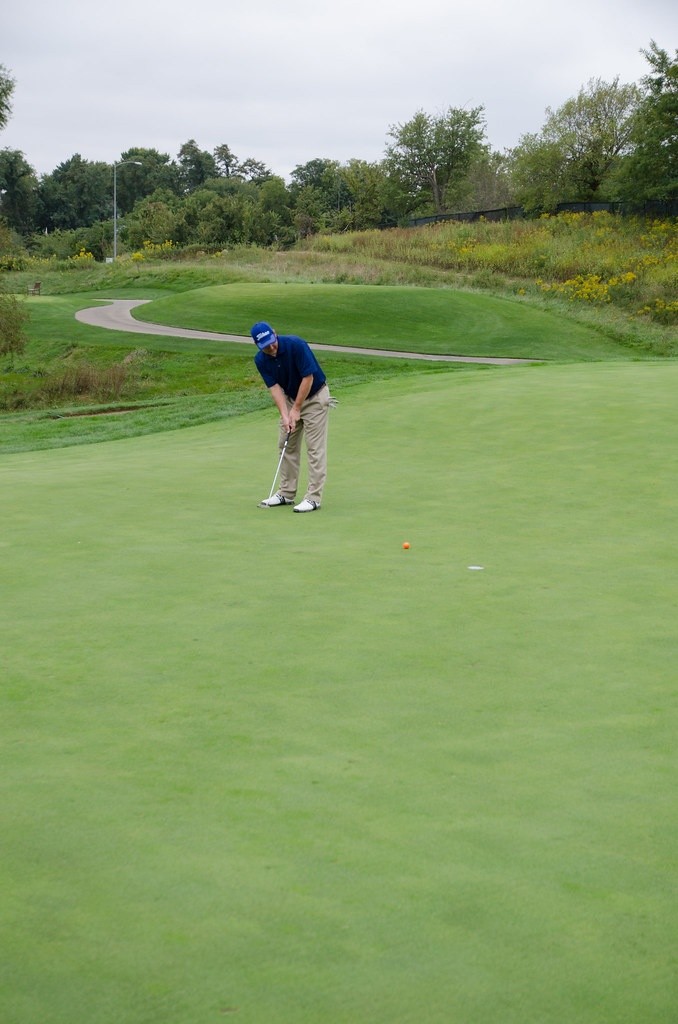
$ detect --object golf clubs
[257,431,291,509]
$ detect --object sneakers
[294,498,321,512]
[261,493,295,507]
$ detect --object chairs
[27,282,41,296]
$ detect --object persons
[251,322,330,513]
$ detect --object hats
[251,322,276,349]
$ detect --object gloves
[328,397,339,408]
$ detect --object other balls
[403,543,409,549]
